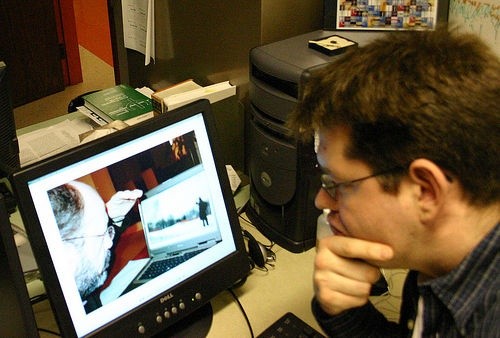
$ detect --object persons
[196,197,210,226]
[47,181,143,316]
[311,26,500,338]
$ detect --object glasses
[65,225,116,241]
[320,167,453,200]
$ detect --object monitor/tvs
[10,99,249,338]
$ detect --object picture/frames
[326,0,452,30]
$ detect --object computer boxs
[244,27,390,254]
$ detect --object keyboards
[256,312,326,338]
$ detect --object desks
[16,79,413,338]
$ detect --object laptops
[119,164,223,299]
